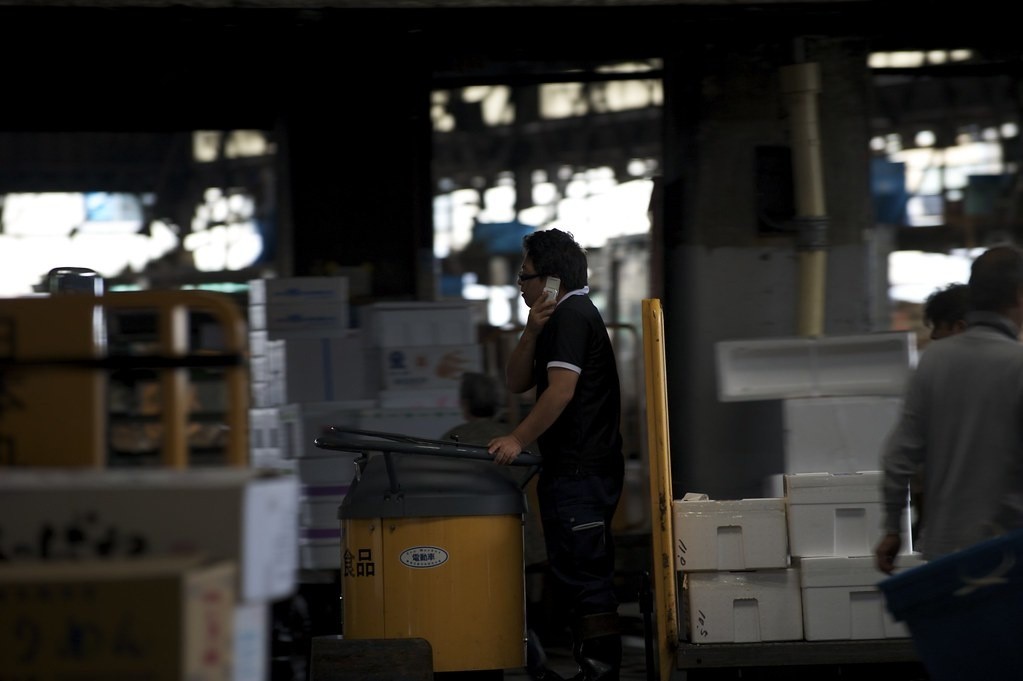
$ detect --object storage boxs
[671,497,792,571]
[0,276,488,681]
[782,394,905,473]
[783,470,914,557]
[715,331,918,401]
[800,550,928,640]
[688,567,804,643]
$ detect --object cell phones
[543,277,560,302]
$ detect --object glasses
[518,271,540,282]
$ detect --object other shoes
[577,658,620,681]
[527,611,583,657]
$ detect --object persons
[438,373,547,567]
[487,228,624,681]
[923,285,975,341]
[876,246,1023,577]
